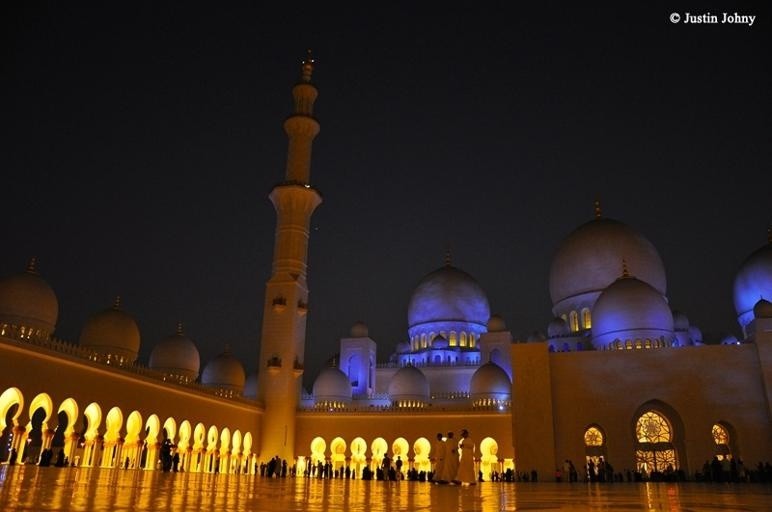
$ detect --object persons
[255,451,432,482]
[39,449,68,467]
[444,432,457,485]
[160,439,180,473]
[490,458,771,480]
[450,430,477,486]
[429,433,445,485]
